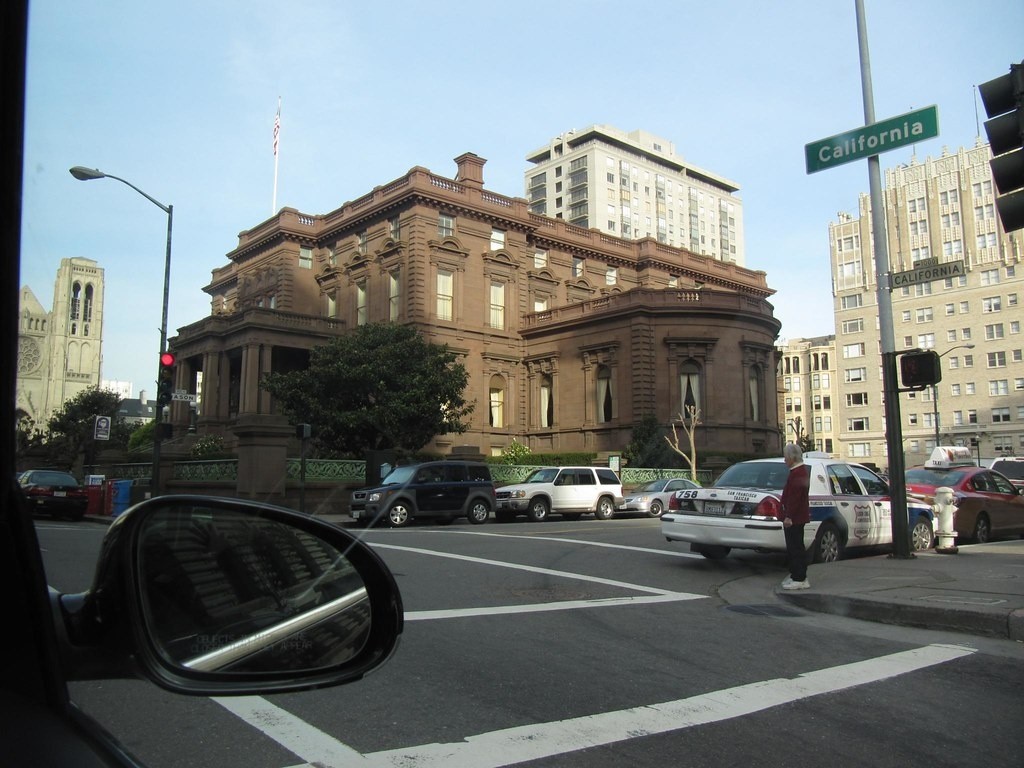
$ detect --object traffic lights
[156,351,176,408]
[901,349,943,390]
[978,61,1024,233]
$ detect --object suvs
[493,466,625,519]
[346,459,496,528]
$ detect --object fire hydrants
[933,485,960,552]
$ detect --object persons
[777,443,810,590]
[396,459,409,469]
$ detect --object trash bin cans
[130,478,151,507]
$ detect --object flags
[273,111,280,155]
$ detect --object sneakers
[783,578,809,589]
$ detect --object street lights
[68,167,173,493]
[975,435,981,467]
[933,344,975,447]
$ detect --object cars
[141,511,370,674]
[659,445,940,563]
[887,444,1024,543]
[614,478,705,517]
[989,456,1024,493]
[19,470,88,521]
